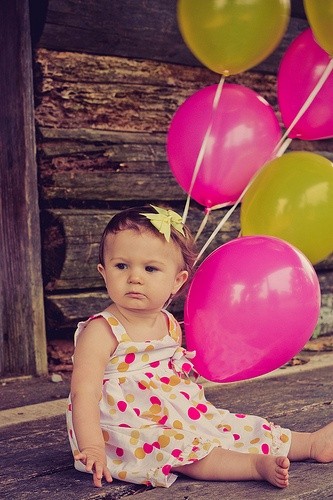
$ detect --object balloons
[176,1,290,77]
[304,0,333,57]
[166,83,281,212]
[184,237,321,385]
[239,151,332,265]
[277,30,333,140]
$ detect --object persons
[66,207,333,487]
